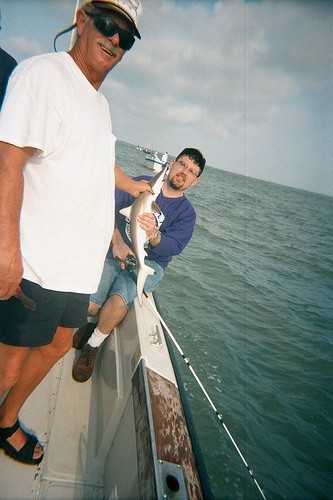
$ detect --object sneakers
[72,341,99,382]
[73,321,98,349]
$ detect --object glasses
[87,12,135,51]
[177,159,199,179]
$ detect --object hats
[83,0,143,41]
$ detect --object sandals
[0,418,44,464]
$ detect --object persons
[0,0,154,466]
[72,148,206,383]
